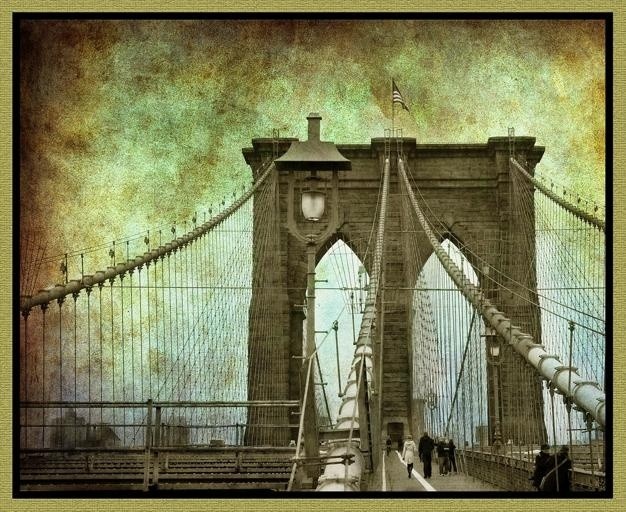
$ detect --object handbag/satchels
[539,476,549,490]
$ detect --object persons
[417,431,435,478]
[448,439,458,473]
[386,438,392,456]
[443,441,451,473]
[402,436,417,477]
[398,436,403,452]
[436,438,450,476]
[544,445,572,492]
[532,443,549,489]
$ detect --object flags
[391,82,411,114]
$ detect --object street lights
[277,111,351,483]
[479,327,504,456]
